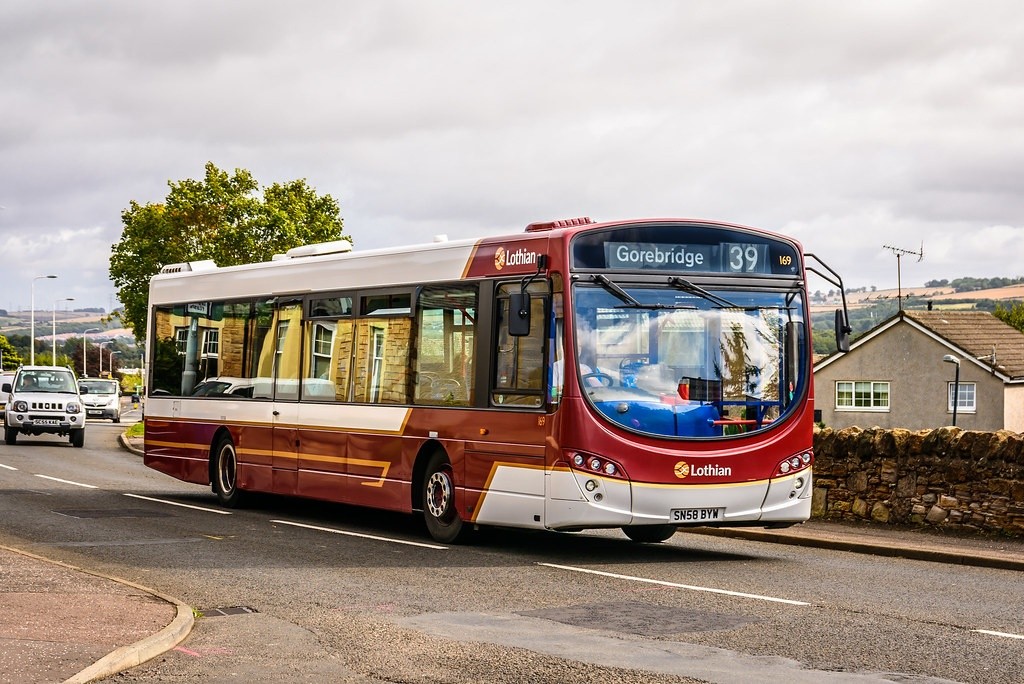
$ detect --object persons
[23,374,35,387]
[553,337,605,394]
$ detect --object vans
[76,377,123,423]
[190,376,336,402]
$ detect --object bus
[144,218,851,543]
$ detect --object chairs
[421,371,460,400]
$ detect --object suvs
[0,363,88,447]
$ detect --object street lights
[109,350,122,378]
[30,275,58,366]
[100,341,114,372]
[83,328,100,378]
[52,297,75,366]
[942,354,961,427]
